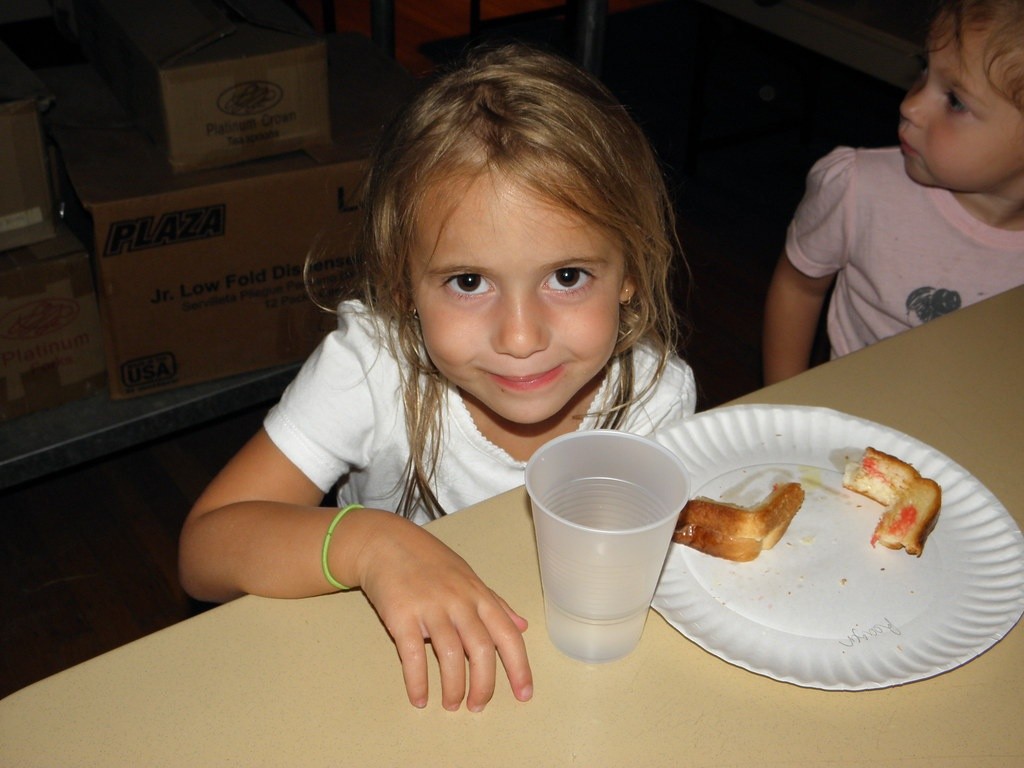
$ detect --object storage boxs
[0,0,392,406]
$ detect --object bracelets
[322,504,365,591]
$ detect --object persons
[181,46,695,713]
[763,0,1024,387]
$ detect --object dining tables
[0,286,1023,766]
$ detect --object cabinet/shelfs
[416,0,936,359]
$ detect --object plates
[645,405,1024,690]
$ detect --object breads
[670,482,806,562]
[840,445,942,558]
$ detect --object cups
[525,429,692,662]
